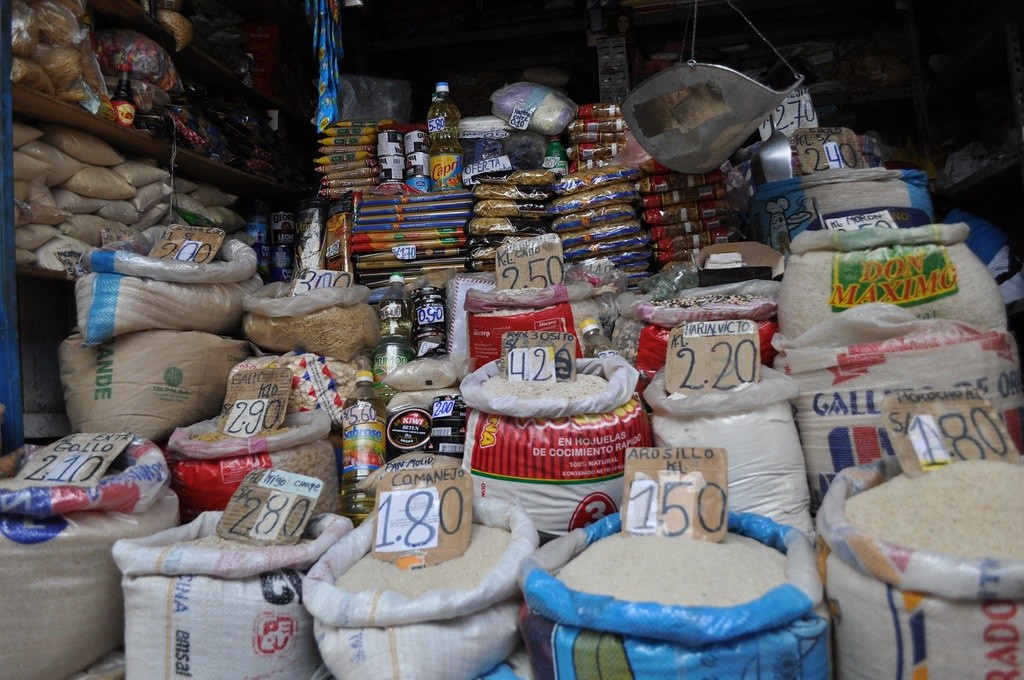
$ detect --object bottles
[245,197,270,285]
[109,62,138,128]
[426,81,463,193]
[338,369,388,527]
[542,134,570,179]
[371,271,415,399]
[270,196,294,283]
[577,318,621,359]
